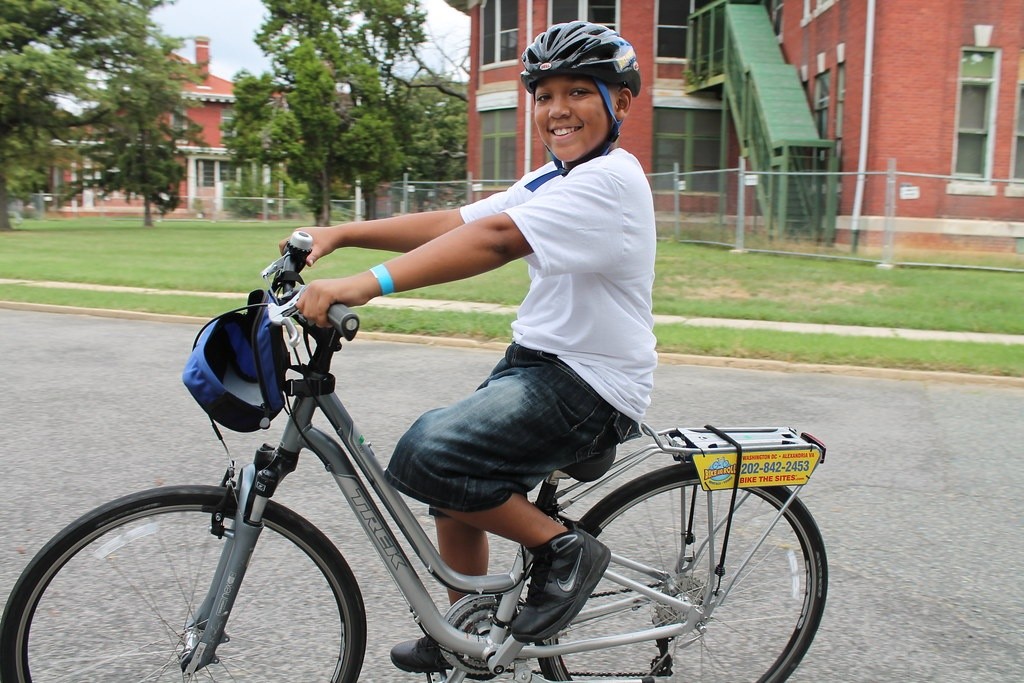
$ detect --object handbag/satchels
[182,287,285,433]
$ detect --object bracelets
[369,264,395,296]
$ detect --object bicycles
[0,230,829,683]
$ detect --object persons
[279,43,656,674]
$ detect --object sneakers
[390,633,501,681]
[509,522,611,643]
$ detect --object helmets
[520,20,642,99]
